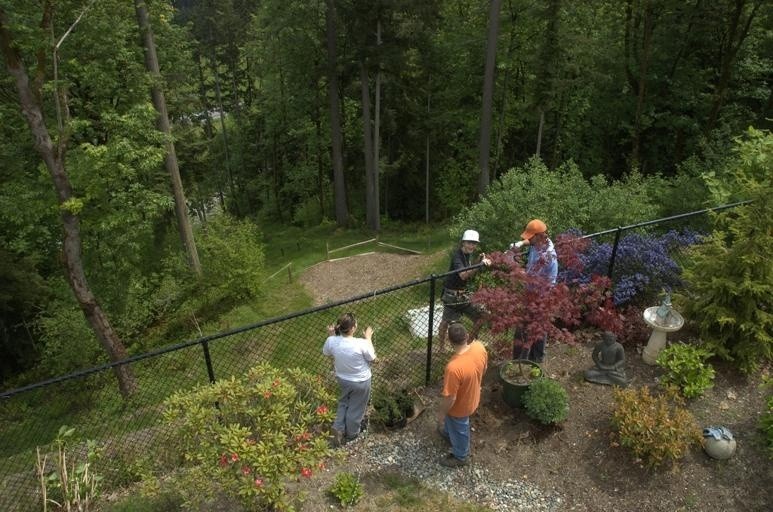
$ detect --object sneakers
[331,426,344,446]
[440,454,466,467]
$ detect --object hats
[520,219,547,239]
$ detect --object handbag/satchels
[441,288,470,308]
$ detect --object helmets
[461,230,480,243]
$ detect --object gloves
[720,426,733,440]
[703,428,721,440]
[481,258,491,267]
[510,241,523,249]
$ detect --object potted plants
[370,379,416,431]
[476,237,623,407]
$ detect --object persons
[509,218,559,374]
[437,323,489,468]
[437,229,493,357]
[323,312,378,452]
